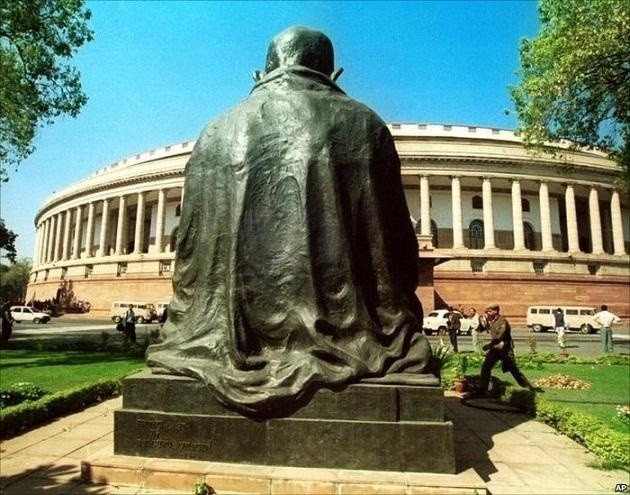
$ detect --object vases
[2,392,22,406]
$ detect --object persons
[23,281,90,315]
[143,24,433,418]
[161,304,169,324]
[446,307,460,353]
[592,304,622,352]
[468,307,480,351]
[125,304,138,343]
[478,304,534,395]
[1,299,14,344]
[554,307,568,349]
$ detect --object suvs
[4,305,51,324]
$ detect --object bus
[526,305,603,335]
[155,302,171,319]
[110,301,158,325]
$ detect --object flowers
[1,381,49,400]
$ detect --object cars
[421,309,472,336]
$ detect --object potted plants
[559,334,569,360]
[527,335,537,354]
[453,354,468,393]
